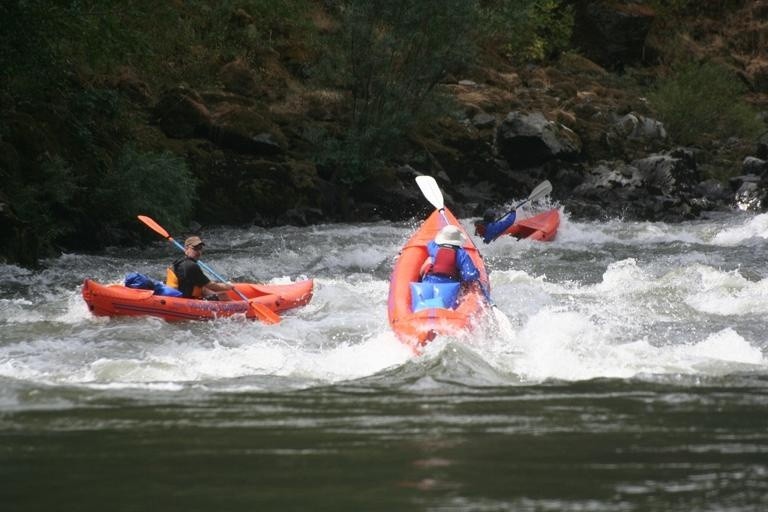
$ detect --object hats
[184,236,205,247]
[434,225,463,249]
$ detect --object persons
[475,207,517,244]
[419,225,481,284]
[166,235,233,302]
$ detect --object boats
[389,199,490,352]
[493,205,561,244]
[82,275,315,323]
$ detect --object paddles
[415,175,514,340]
[494,179,553,223]
[138,215,282,325]
